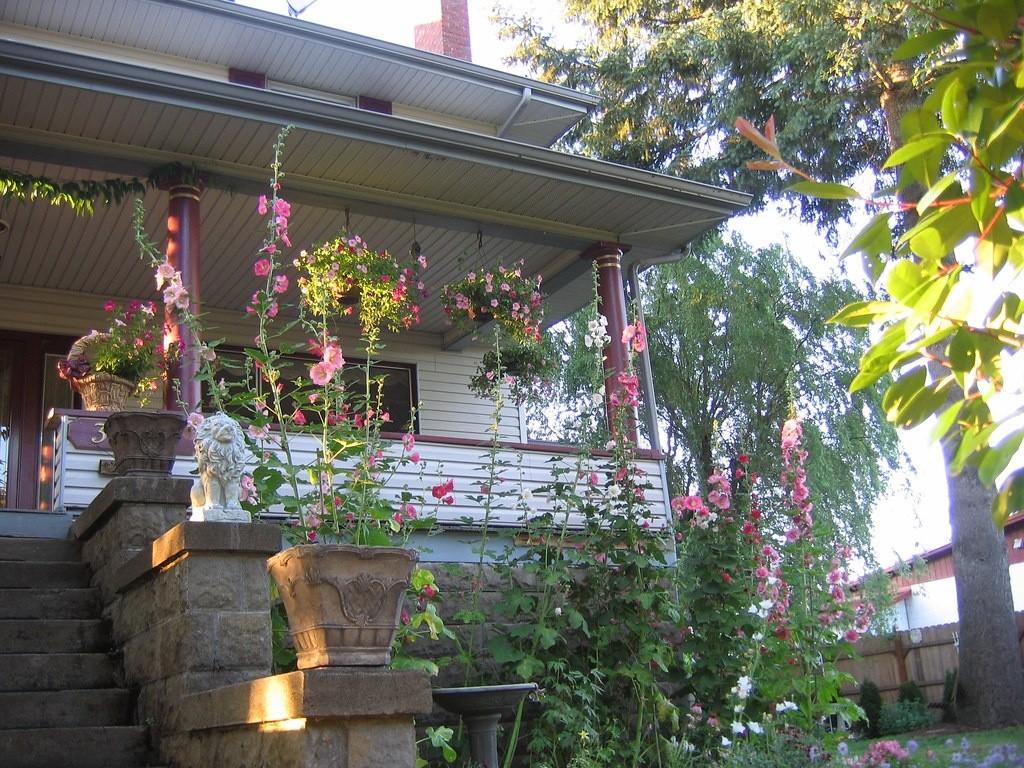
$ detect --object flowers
[87,300,188,409]
[440,250,558,345]
[292,223,429,341]
[466,336,563,409]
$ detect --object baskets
[65,331,142,412]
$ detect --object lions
[190,411,245,511]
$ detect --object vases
[266,542,421,669]
[335,283,364,305]
[465,308,495,322]
[103,412,188,475]
[503,364,520,376]
